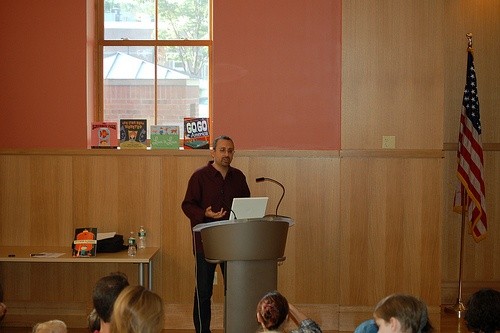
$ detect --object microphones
[255,177,286,215]
[221,189,237,220]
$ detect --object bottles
[128,231,137,256]
[138,226,147,249]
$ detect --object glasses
[214,148,235,154]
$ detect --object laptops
[229,198,268,220]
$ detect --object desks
[0,246,161,292]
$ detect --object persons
[373,294,428,333]
[181,136,251,333]
[256,292,323,333]
[32,319,67,333]
[87,272,129,333]
[110,285,163,333]
[462,287,500,333]
